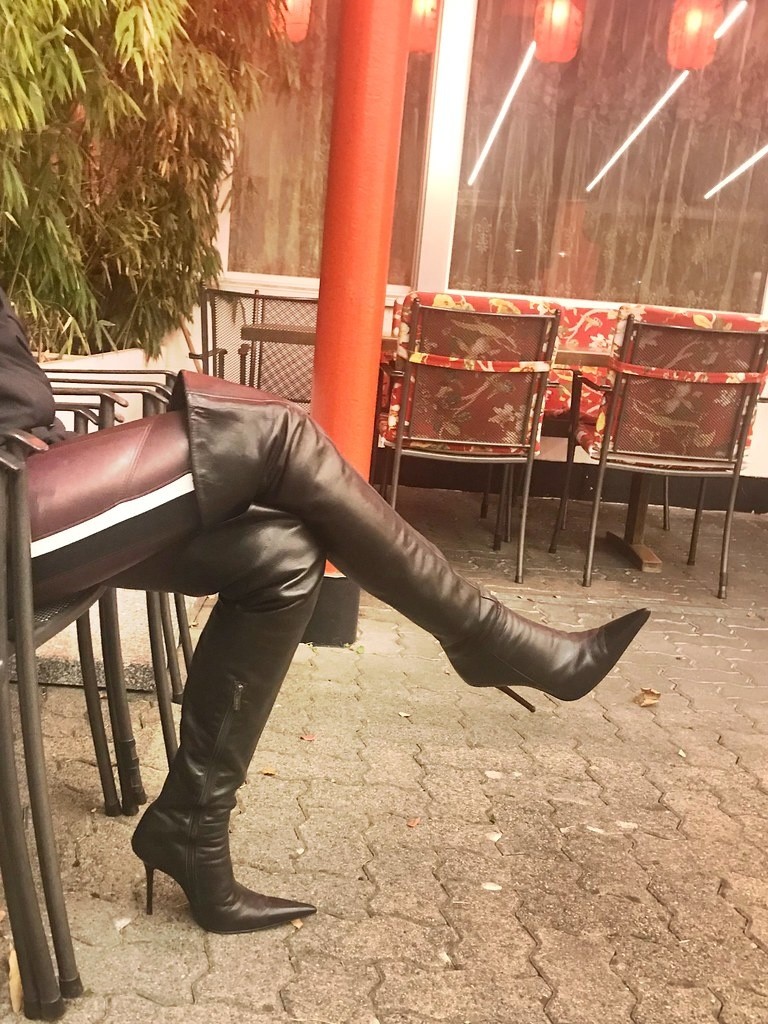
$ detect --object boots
[173,369,651,713]
[131,510,325,933]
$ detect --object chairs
[189,289,768,598]
[0,369,193,1019]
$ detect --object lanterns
[407,0,441,53]
[266,0,311,42]
[531,1,585,64]
[668,1,724,71]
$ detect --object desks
[242,325,663,572]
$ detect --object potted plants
[1,1,298,434]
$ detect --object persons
[0,303,651,936]
